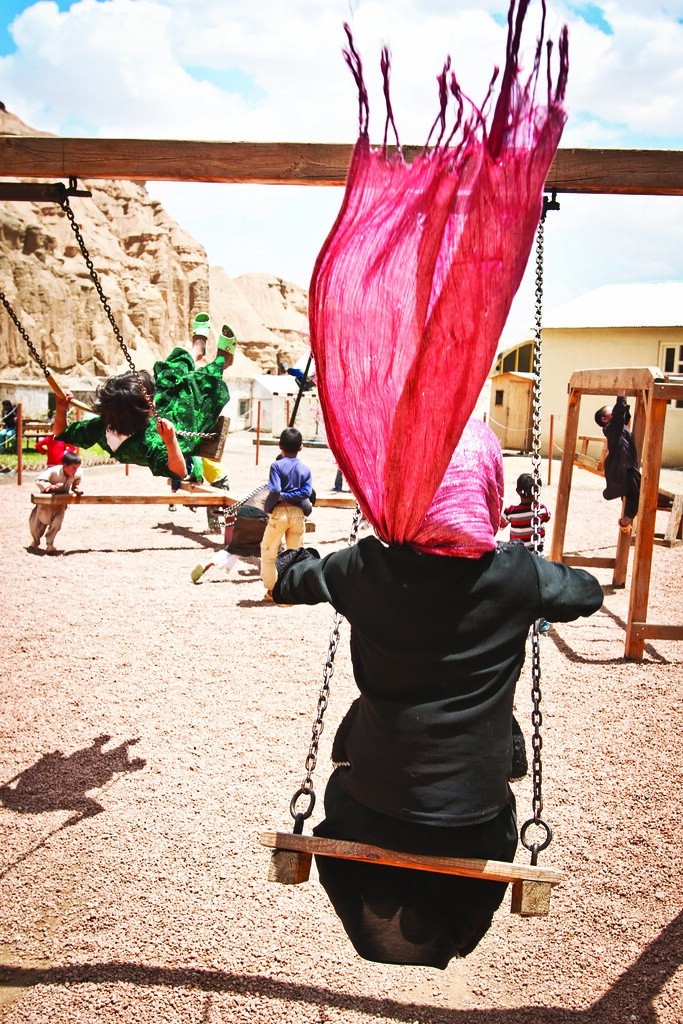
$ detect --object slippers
[192,312,211,356]
[218,325,237,366]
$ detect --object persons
[499,473,550,636]
[272,418,605,966]
[53,312,238,481]
[36,423,75,469]
[0,400,17,453]
[595,389,641,535]
[260,428,313,601]
[29,452,83,551]
[275,452,317,505]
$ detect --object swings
[1,177,233,479]
[253,192,560,917]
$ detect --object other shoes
[46,546,56,553]
[618,519,636,537]
[31,541,40,549]
[538,619,549,631]
[263,591,274,601]
